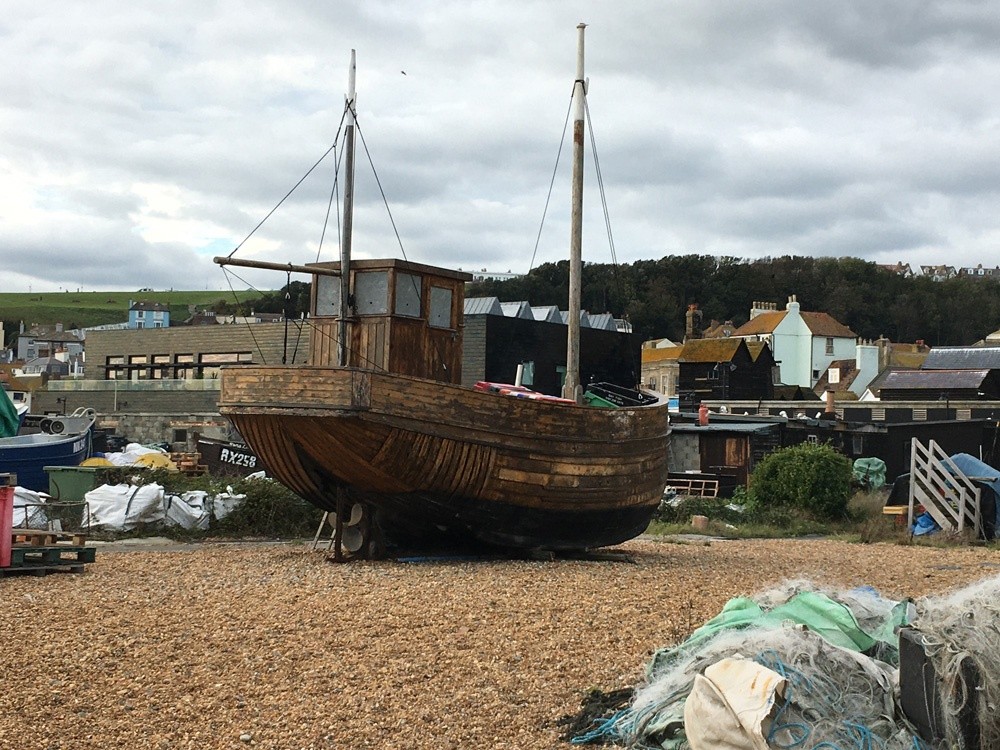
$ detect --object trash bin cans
[43,466,96,501]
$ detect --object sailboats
[212,22,673,564]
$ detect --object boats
[0,400,98,497]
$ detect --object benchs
[12,546,96,564]
[13,528,85,546]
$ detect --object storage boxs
[473,381,658,407]
